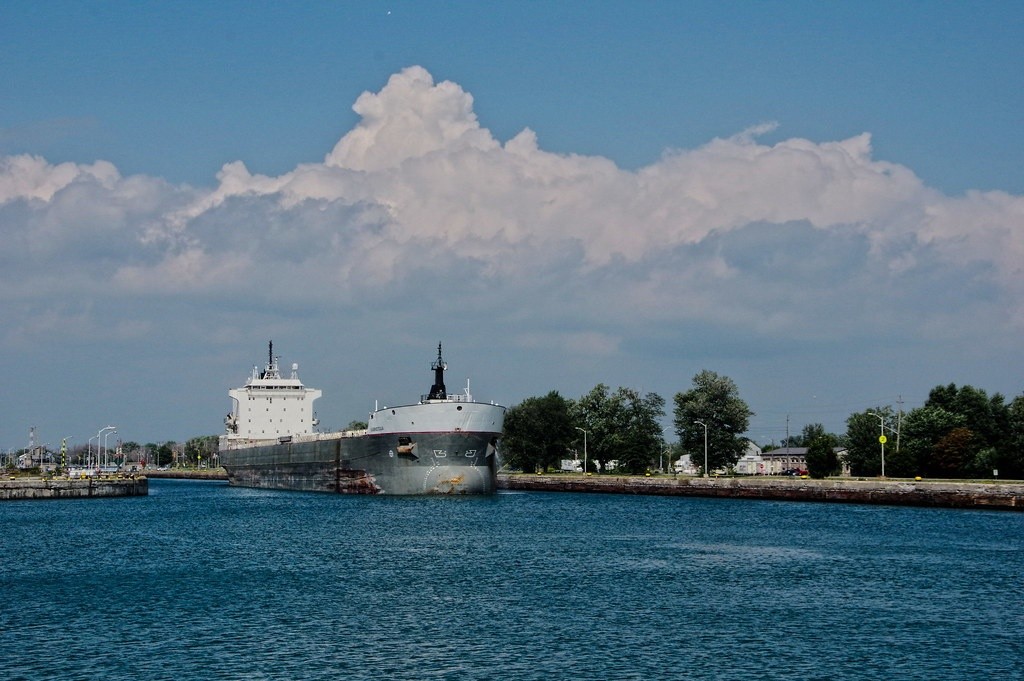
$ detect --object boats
[218,338,506,495]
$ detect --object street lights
[575,427,586,475]
[88,435,99,469]
[868,413,884,477]
[98,427,116,468]
[104,431,117,469]
[41,443,50,472]
[694,421,707,478]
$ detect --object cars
[779,468,808,476]
